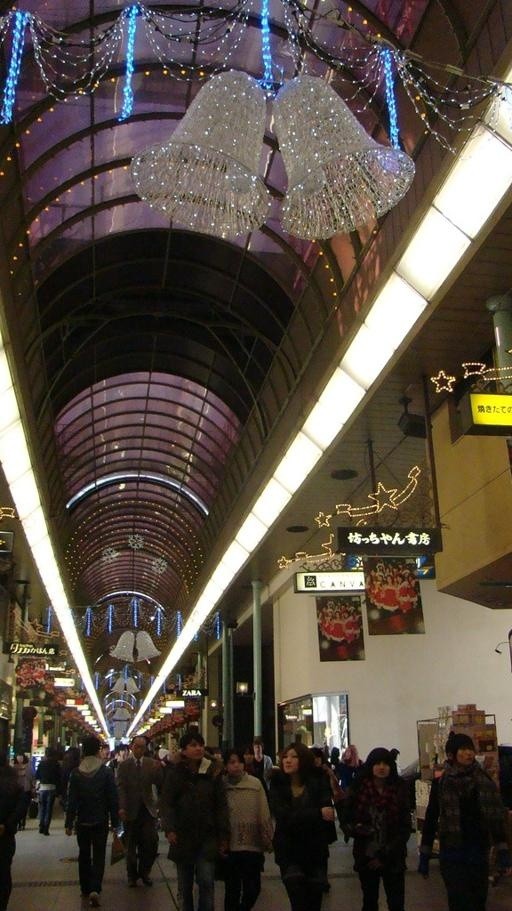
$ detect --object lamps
[123,60,420,250]
[109,592,164,723]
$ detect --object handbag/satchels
[111,835,125,865]
[29,802,39,818]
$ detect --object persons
[12,747,66,836]
[1,731,24,909]
[269,741,412,910]
[317,596,364,645]
[417,734,512,910]
[365,558,420,615]
[66,732,274,910]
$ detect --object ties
[136,759,141,771]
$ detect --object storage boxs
[412,700,505,880]
